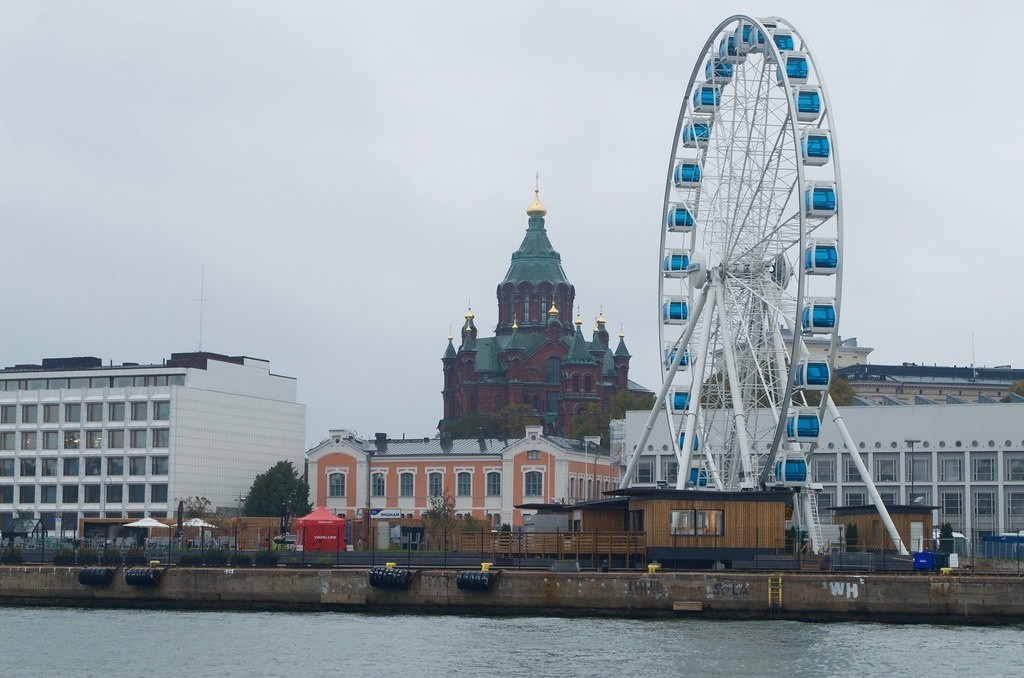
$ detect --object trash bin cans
[913,552,935,571]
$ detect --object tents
[296,506,346,551]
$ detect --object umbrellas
[122,517,173,538]
[172,518,219,548]
